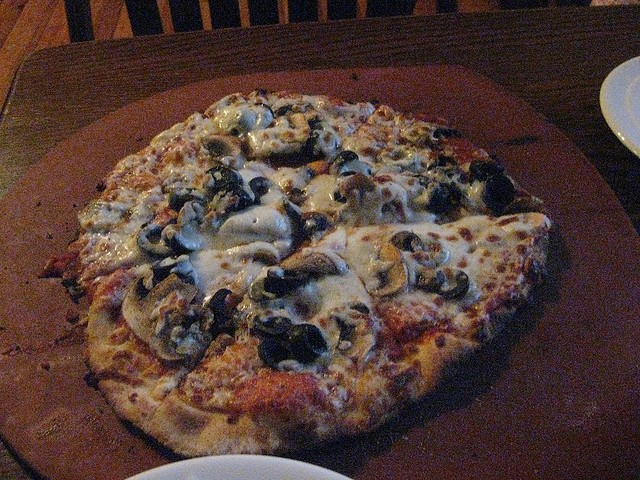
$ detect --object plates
[599,56,640,157]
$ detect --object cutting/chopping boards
[0,65,640,479]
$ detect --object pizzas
[34,87,554,457]
